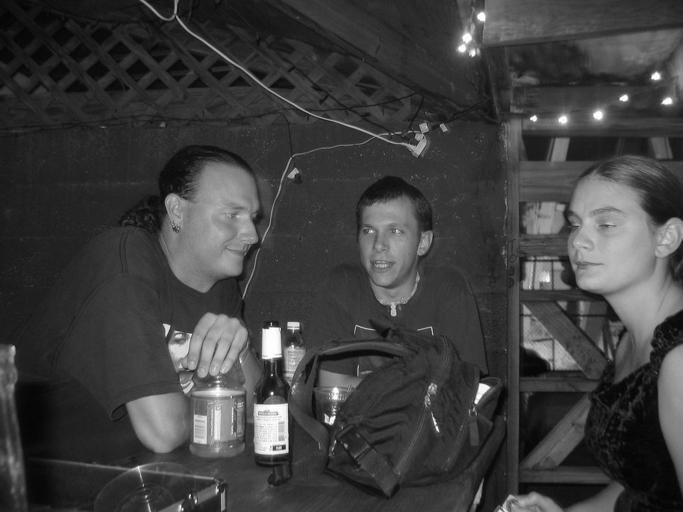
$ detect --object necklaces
[374,287,417,317]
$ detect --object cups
[312,385,355,429]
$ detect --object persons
[11,144,262,509]
[301,175,489,386]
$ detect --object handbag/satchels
[287,313,504,498]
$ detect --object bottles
[284,320,306,389]
[189,341,246,460]
[253,322,295,468]
